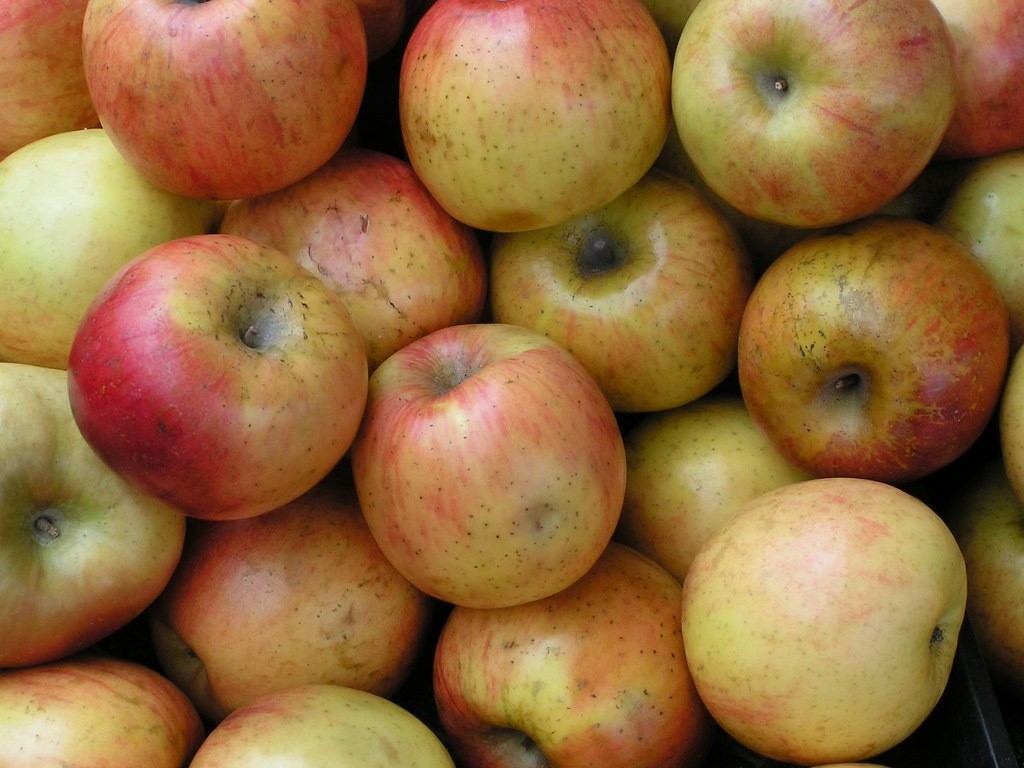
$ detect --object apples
[0,0,1024,768]
